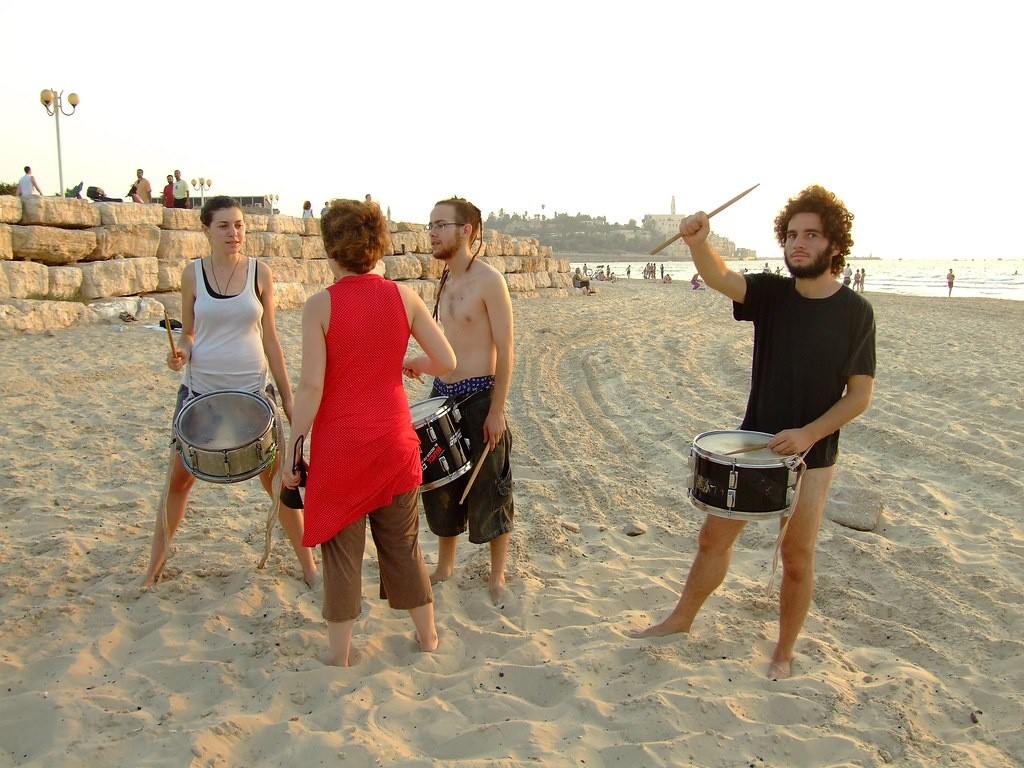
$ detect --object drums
[686,429,797,520]
[408,395,475,494]
[174,388,280,485]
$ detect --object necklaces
[210,256,241,295]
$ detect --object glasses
[423,222,465,232]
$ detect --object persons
[280,200,458,667]
[691,273,700,290]
[366,195,371,200]
[860,268,866,292]
[573,268,595,295]
[641,263,656,279]
[663,274,672,283]
[583,263,587,276]
[16,166,42,195]
[842,263,852,287]
[775,267,784,274]
[420,198,514,603]
[321,202,329,217]
[947,269,955,297]
[592,265,616,282]
[763,263,771,273]
[660,264,663,280]
[134,169,152,203]
[630,186,877,677]
[302,201,314,218]
[626,265,630,277]
[853,269,861,291]
[143,196,317,589]
[163,170,190,210]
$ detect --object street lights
[265,193,280,215]
[191,177,212,209]
[40,88,80,198]
